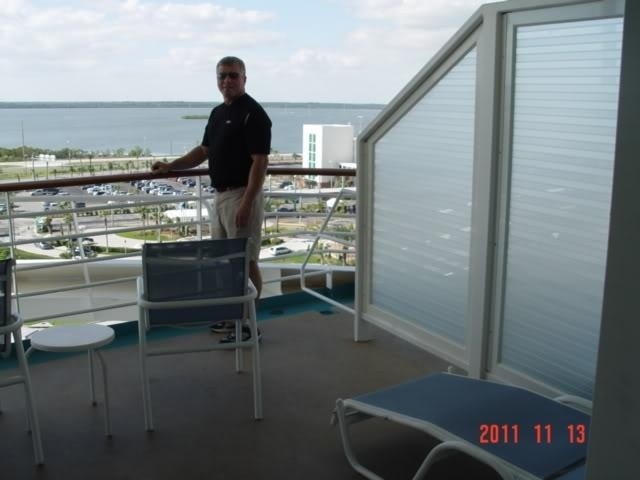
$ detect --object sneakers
[208,318,263,344]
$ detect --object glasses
[218,71,243,81]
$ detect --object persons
[151,56,272,344]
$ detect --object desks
[18,321,119,445]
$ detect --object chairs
[325,362,592,480]
[131,233,267,432]
[1,256,46,468]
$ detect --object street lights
[66,150,155,177]
[38,153,56,180]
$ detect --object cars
[42,201,89,210]
[72,246,95,259]
[262,181,300,211]
[105,199,202,210]
[0,203,7,214]
[80,178,215,197]
[31,189,60,196]
[36,241,50,250]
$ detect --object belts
[212,186,244,193]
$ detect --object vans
[269,245,291,256]
